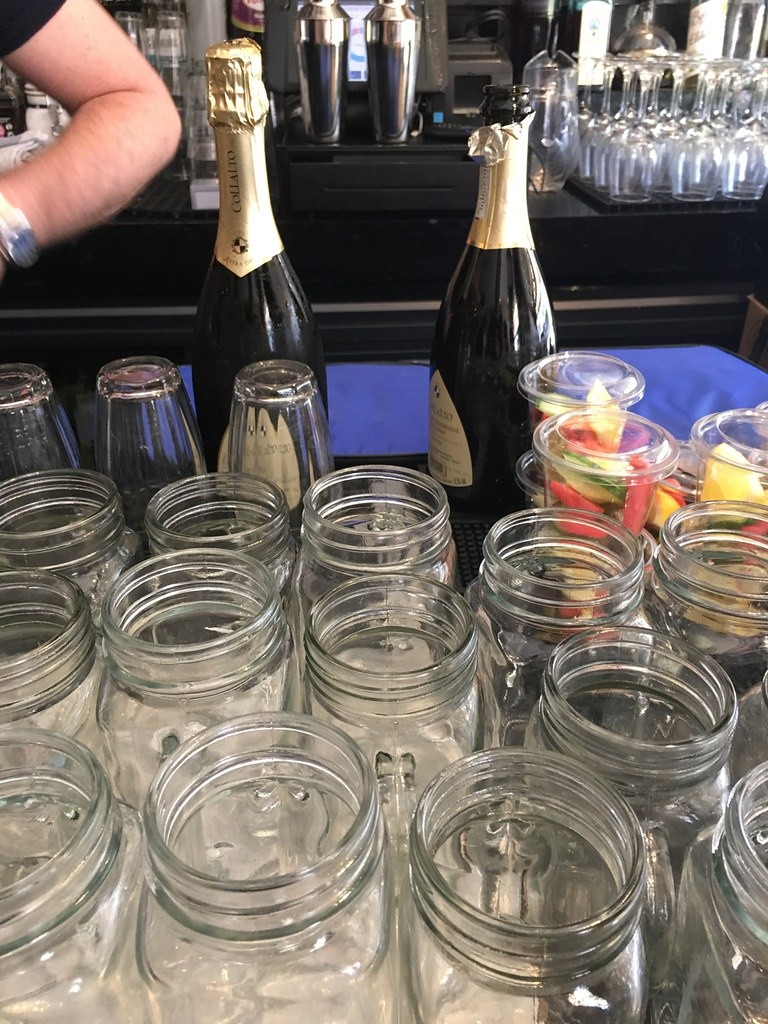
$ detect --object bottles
[1,471,768,1024]
[188,36,327,474]
[430,84,559,515]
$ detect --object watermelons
[552,426,688,537]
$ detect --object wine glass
[522,0,768,203]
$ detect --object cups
[229,359,335,548]
[95,357,208,558]
[155,9,187,122]
[0,363,82,481]
[117,12,148,62]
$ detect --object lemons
[647,491,682,530]
[700,442,766,506]
[586,379,625,448]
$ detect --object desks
[140,340,768,712]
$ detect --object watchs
[0,193,40,268]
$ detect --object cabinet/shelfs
[0,149,768,365]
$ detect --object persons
[0,0,182,268]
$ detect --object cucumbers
[555,462,624,504]
[536,393,578,417]
[560,451,626,494]
